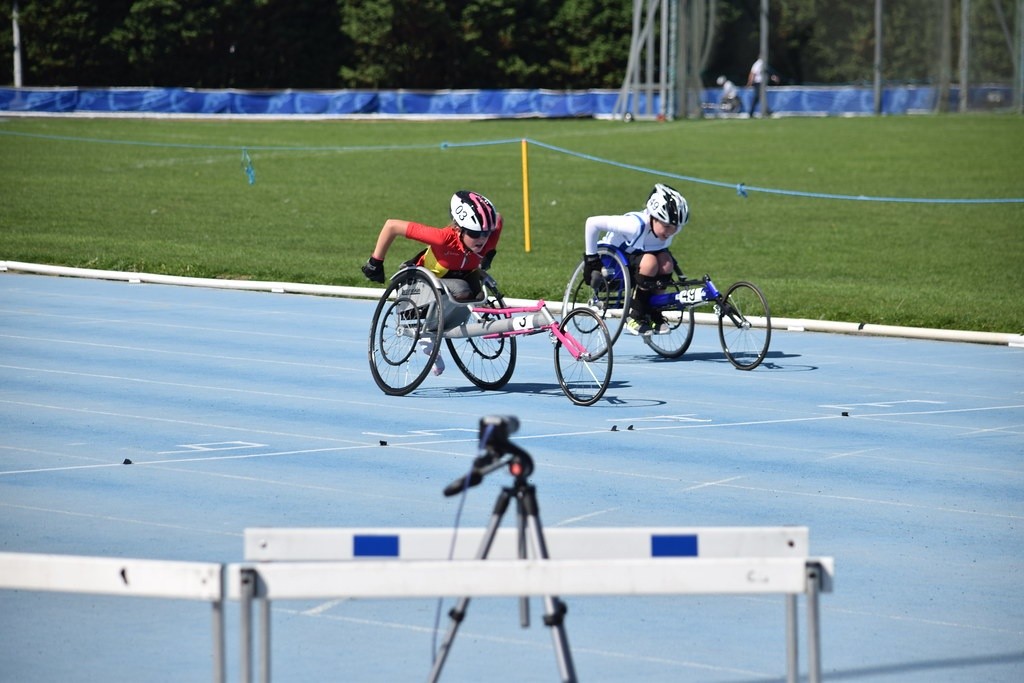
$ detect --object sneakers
[645,317,671,334]
[624,316,654,336]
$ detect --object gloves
[583,252,605,286]
[481,249,498,270]
[362,256,386,284]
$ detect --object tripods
[427,442,579,683]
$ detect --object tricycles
[368,268,613,407]
[560,249,772,374]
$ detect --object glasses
[463,228,491,239]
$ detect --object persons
[361,190,504,376]
[746,54,778,120]
[717,75,743,113]
[585,182,689,335]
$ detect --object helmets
[647,183,689,225]
[450,190,497,232]
